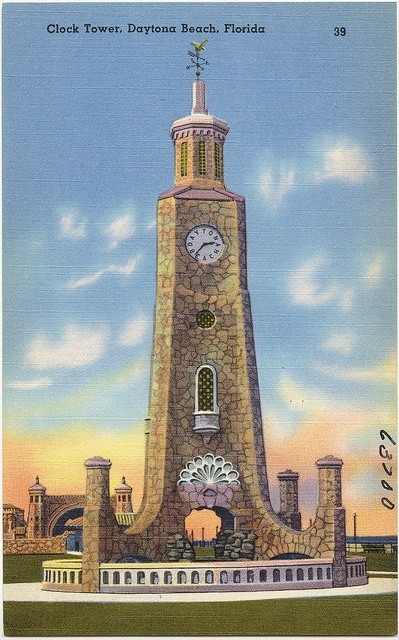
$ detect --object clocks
[185,223,224,264]
